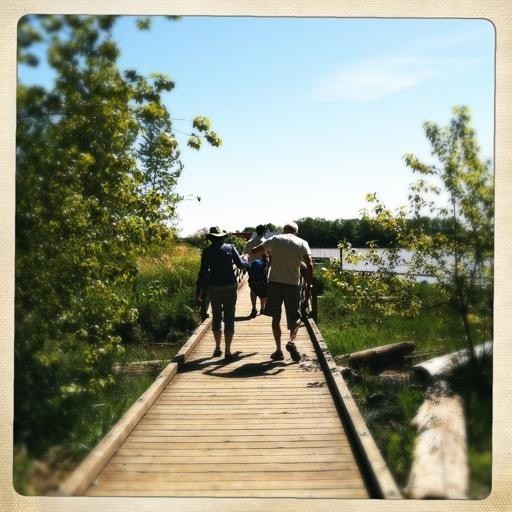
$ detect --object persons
[196,225,252,360]
[249,222,315,363]
[195,264,212,321]
[242,224,270,317]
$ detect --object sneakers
[271,350,284,360]
[250,312,256,318]
[260,309,264,314]
[286,341,301,361]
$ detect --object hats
[205,226,228,237]
[256,225,269,231]
